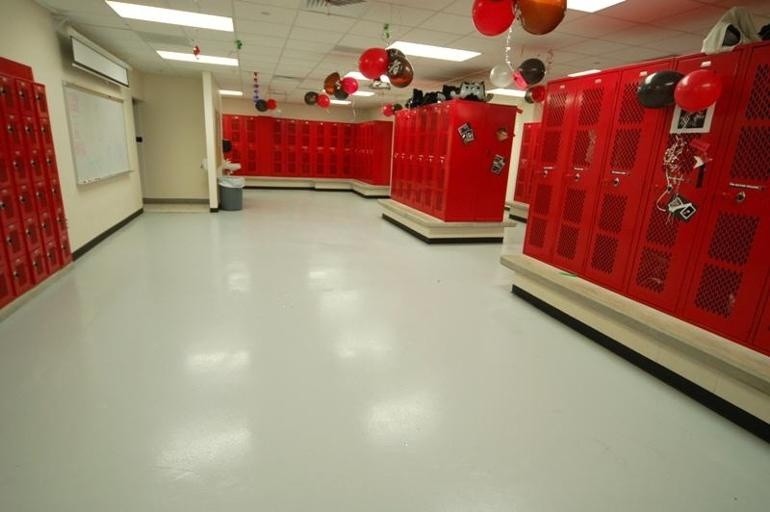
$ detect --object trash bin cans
[218,176,245,211]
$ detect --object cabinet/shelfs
[522,41,770,353]
[222,112,393,186]
[513,122,540,205]
[0,57,73,311]
[392,99,518,222]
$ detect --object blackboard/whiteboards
[62,80,135,186]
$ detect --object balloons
[516,0,567,36]
[267,100,277,109]
[488,59,545,103]
[256,99,267,111]
[638,70,684,107]
[674,70,721,111]
[472,1,516,36]
[304,49,414,116]
